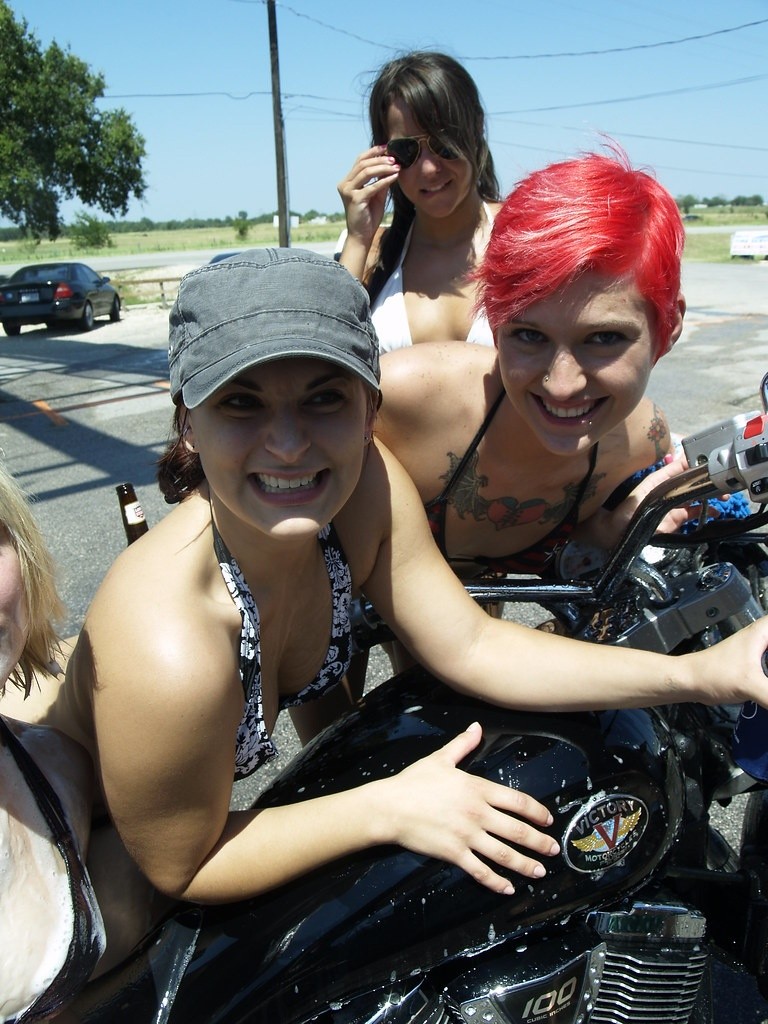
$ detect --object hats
[167,246,383,411]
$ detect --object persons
[1,252,768,1024]
[338,48,520,350]
[295,143,737,747]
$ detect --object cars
[0,263,121,336]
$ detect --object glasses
[385,127,470,169]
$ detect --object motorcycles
[26,373,768,1024]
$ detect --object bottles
[116,482,150,547]
